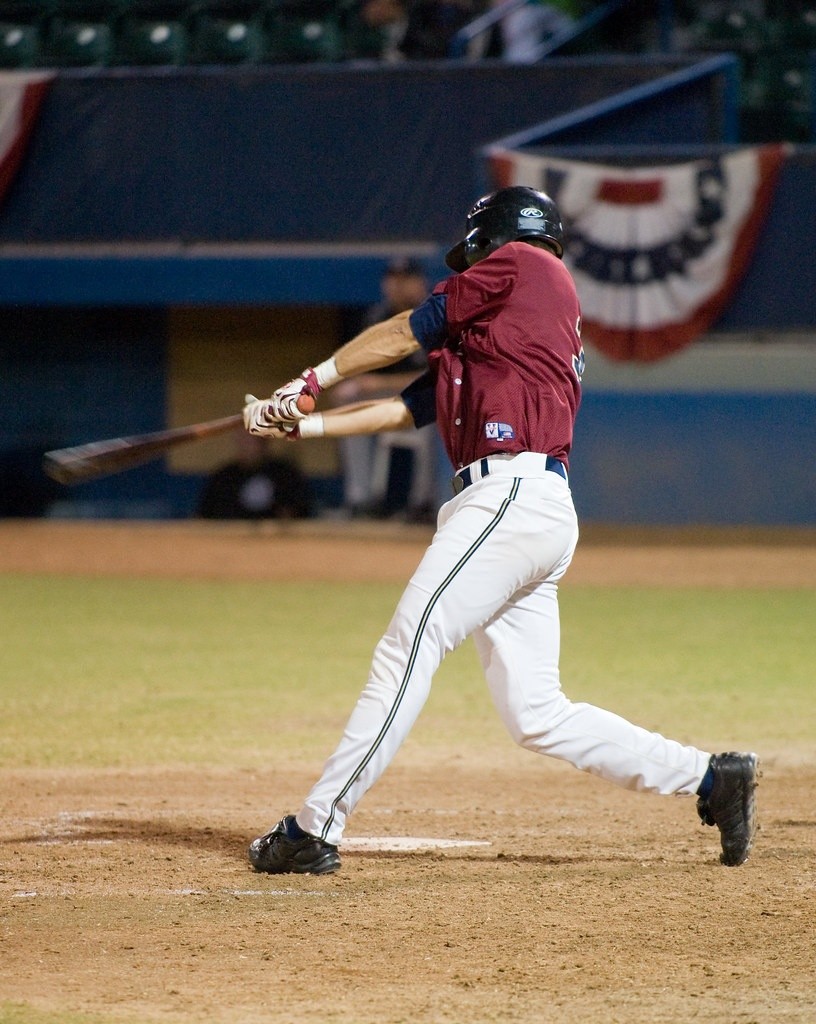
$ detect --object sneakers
[696,751,757,866]
[249,815,343,875]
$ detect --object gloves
[264,355,344,431]
[242,394,324,441]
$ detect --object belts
[452,454,567,495]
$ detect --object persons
[243,187,759,875]
[333,254,438,522]
[341,0,579,62]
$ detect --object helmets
[446,185,565,273]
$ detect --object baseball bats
[46,394,315,489]
